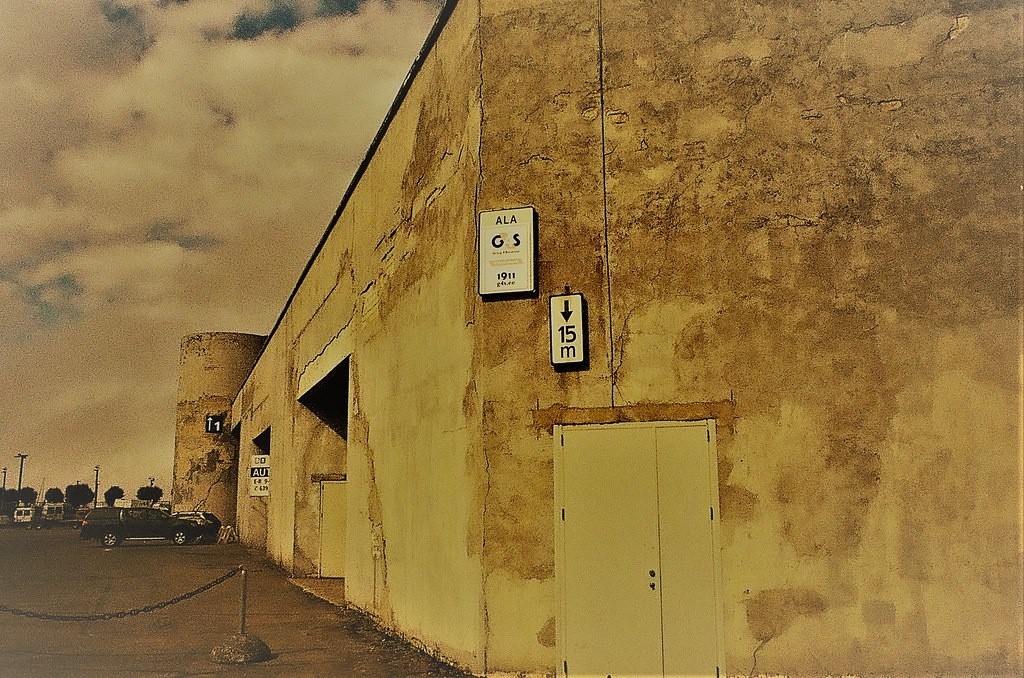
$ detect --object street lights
[3,453,156,508]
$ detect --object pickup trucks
[81,506,199,547]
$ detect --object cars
[170,511,221,536]
